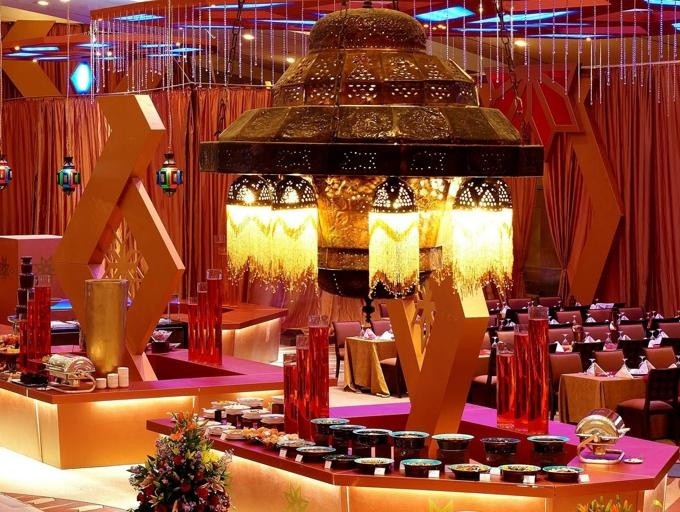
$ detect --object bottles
[282,314,330,442]
[497,307,548,435]
[18,268,224,372]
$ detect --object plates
[206,425,316,449]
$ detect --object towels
[364,326,392,339]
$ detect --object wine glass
[492,302,680,364]
[359,325,365,337]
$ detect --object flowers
[576,492,665,512]
[126,414,234,512]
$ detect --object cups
[96,367,129,389]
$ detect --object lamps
[200,0,545,301]
[55,0,80,194]
[0,34,12,191]
[154,1,183,196]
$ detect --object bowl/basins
[151,331,172,342]
[202,394,283,424]
[297,417,584,481]
